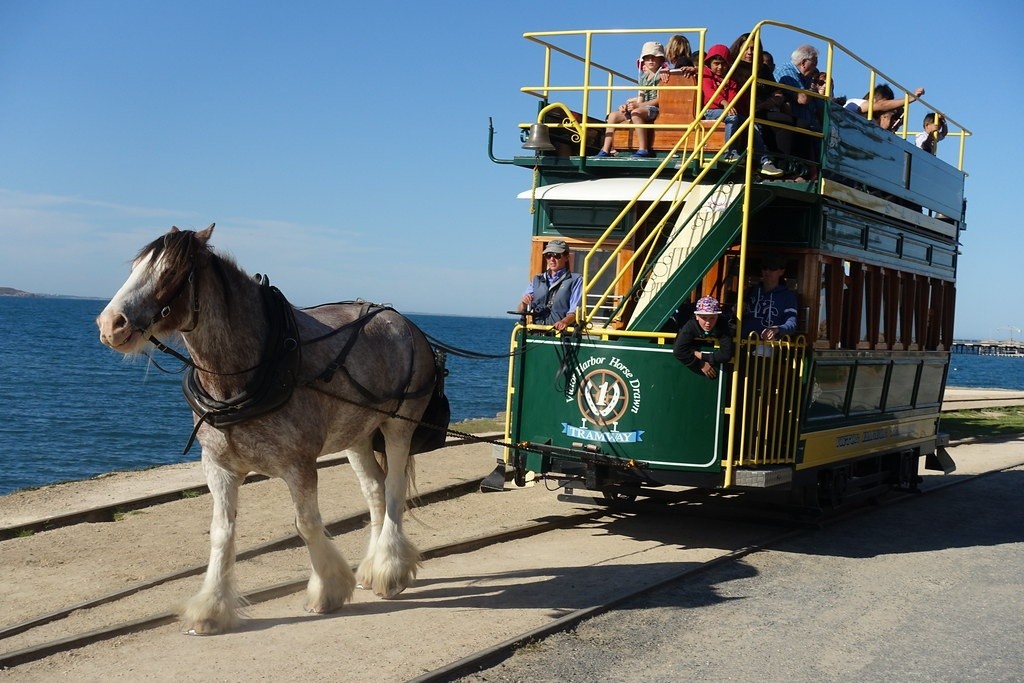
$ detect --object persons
[741,252,799,342]
[702,44,784,176]
[674,296,732,379]
[915,113,948,154]
[656,35,834,182]
[519,240,583,330]
[636,56,646,74]
[595,41,663,157]
[730,33,816,182]
[843,83,925,132]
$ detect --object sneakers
[757,160,783,177]
[723,150,745,164]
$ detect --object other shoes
[597,150,611,157]
[631,150,652,157]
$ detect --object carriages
[95,20,973,638]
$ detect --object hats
[639,41,665,63]
[693,296,722,315]
[542,240,569,254]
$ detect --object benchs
[612,67,739,155]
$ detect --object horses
[95,222,437,635]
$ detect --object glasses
[818,80,825,86]
[544,252,564,259]
